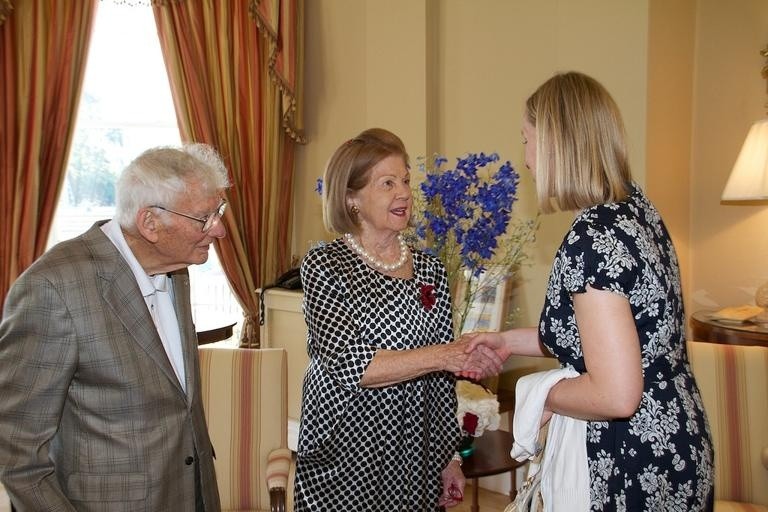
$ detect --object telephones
[276,267,303,289]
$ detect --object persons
[291,126,504,512]
[454,70,716,512]
[0,148,229,512]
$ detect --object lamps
[716,118,768,307]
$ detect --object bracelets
[452,451,464,467]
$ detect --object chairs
[198,346,294,512]
[679,337,767,510]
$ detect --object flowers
[411,148,541,344]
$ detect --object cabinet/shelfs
[250,284,311,424]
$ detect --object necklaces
[345,231,410,273]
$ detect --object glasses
[149,198,228,233]
